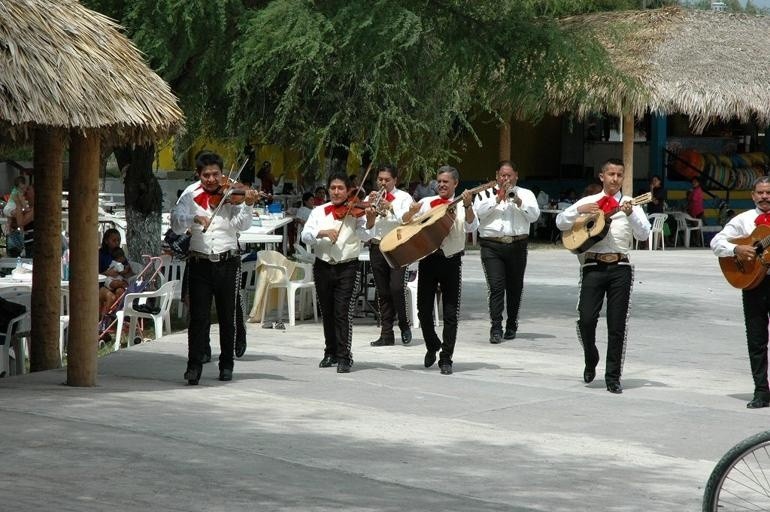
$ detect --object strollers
[98,254,165,350]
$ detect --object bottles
[16,256,23,272]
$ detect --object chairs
[0,181,323,382]
[669,211,705,249]
[635,213,668,251]
[405,258,441,327]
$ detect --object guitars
[562,192,653,255]
[719,225,769,289]
[379,181,498,268]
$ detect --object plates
[253,204,284,221]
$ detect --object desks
[56,189,127,230]
[352,253,382,327]
[236,209,293,291]
[1,255,107,299]
[537,209,565,242]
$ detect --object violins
[334,195,386,219]
[209,176,273,208]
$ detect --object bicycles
[700,427,770,512]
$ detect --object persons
[467,158,541,344]
[400,164,478,375]
[295,187,328,254]
[354,163,418,346]
[99,229,134,322]
[300,167,374,372]
[171,153,258,384]
[709,175,770,406]
[413,168,439,203]
[3,176,35,257]
[685,176,705,220]
[257,161,287,206]
[103,246,131,292]
[556,158,651,393]
[178,150,258,365]
[648,176,667,215]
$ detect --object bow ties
[756,214,770,226]
[195,192,211,210]
[324,204,344,219]
[383,192,395,202]
[431,199,452,208]
[597,196,620,215]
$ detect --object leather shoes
[184,343,246,385]
[490,327,503,343]
[584,366,596,382]
[371,330,452,375]
[337,363,350,373]
[319,356,337,367]
[747,397,769,408]
[606,382,622,394]
[504,329,515,339]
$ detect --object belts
[328,258,357,265]
[585,253,627,264]
[483,234,529,244]
[370,238,380,245]
[191,250,236,262]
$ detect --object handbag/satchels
[6,230,25,257]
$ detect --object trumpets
[506,183,518,200]
